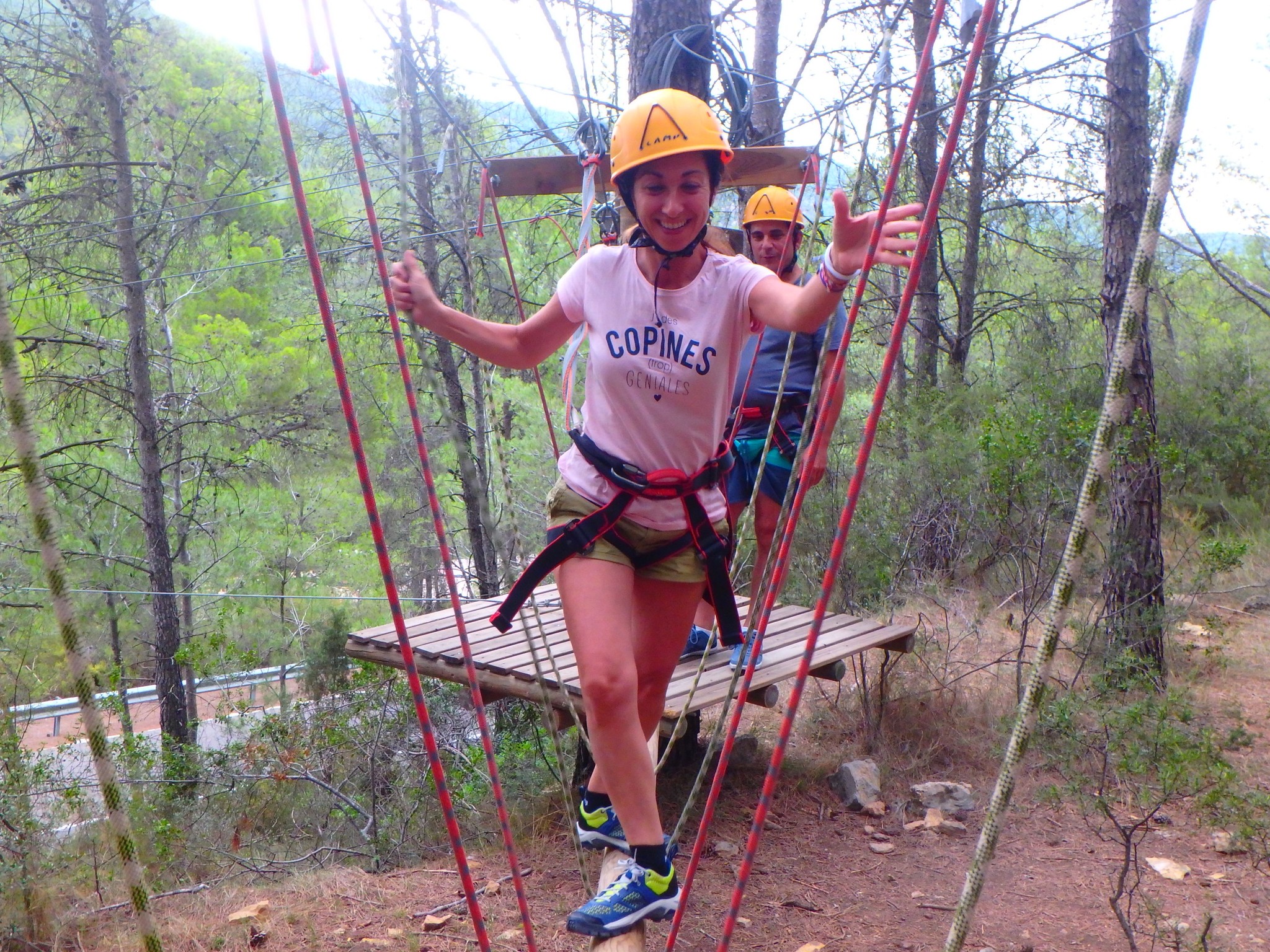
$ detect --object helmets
[610,88,734,188]
[743,186,804,229]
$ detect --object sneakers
[679,624,717,660]
[730,631,763,670]
[576,784,677,859]
[566,848,682,937]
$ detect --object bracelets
[825,242,861,278]
[817,262,849,292]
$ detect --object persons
[679,185,848,670]
[389,88,928,937]
